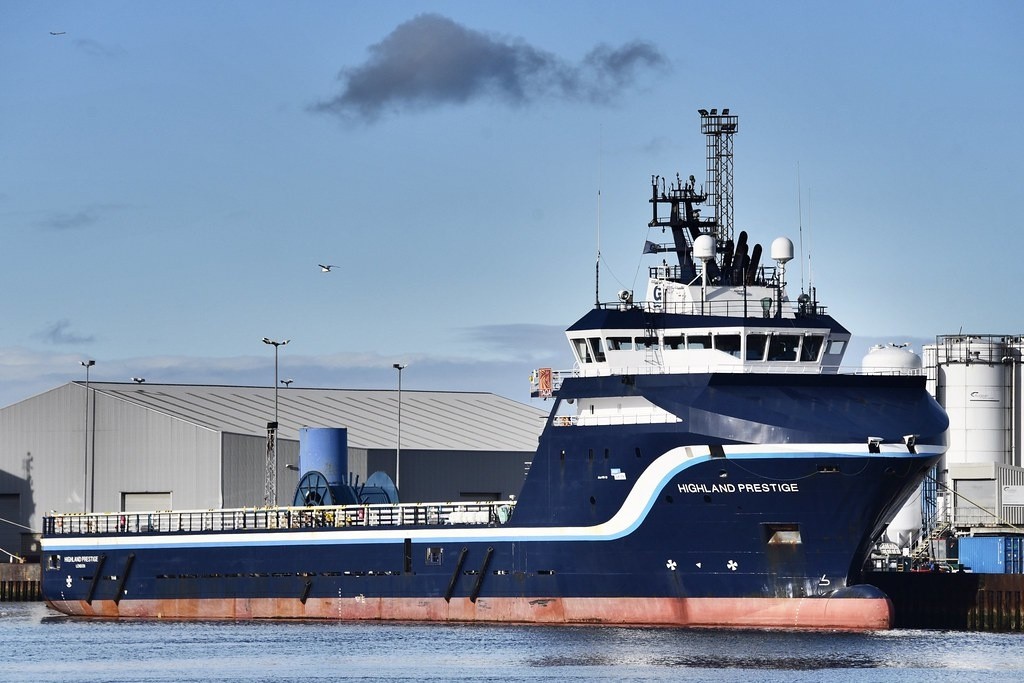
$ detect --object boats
[38,104,952,630]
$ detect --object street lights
[79,359,96,512]
[392,363,404,494]
[262,337,291,508]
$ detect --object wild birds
[318,264,340,272]
